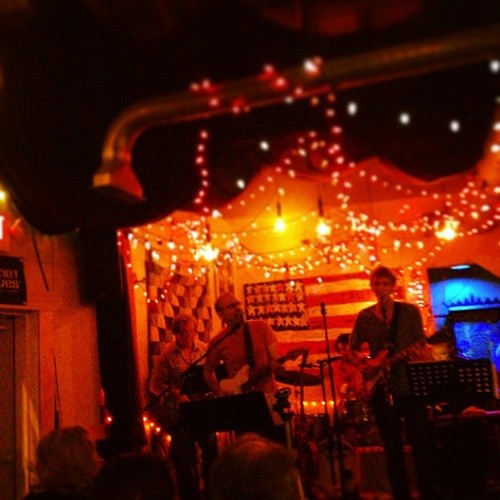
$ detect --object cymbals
[274,370,321,386]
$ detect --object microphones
[231,320,243,332]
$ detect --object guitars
[220,347,304,396]
[352,327,453,400]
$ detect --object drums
[291,413,325,438]
[343,398,374,419]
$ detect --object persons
[333,332,377,405]
[150,311,215,500]
[351,264,426,500]
[202,291,281,434]
[24,425,299,500]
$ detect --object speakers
[355,446,418,489]
[417,407,500,499]
